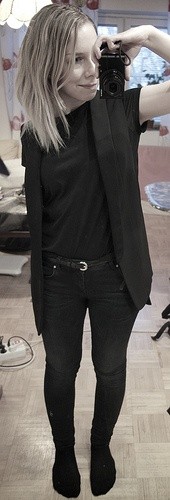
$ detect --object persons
[16,4,170,498]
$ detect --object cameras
[98,49,125,99]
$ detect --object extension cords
[0,339,26,362]
